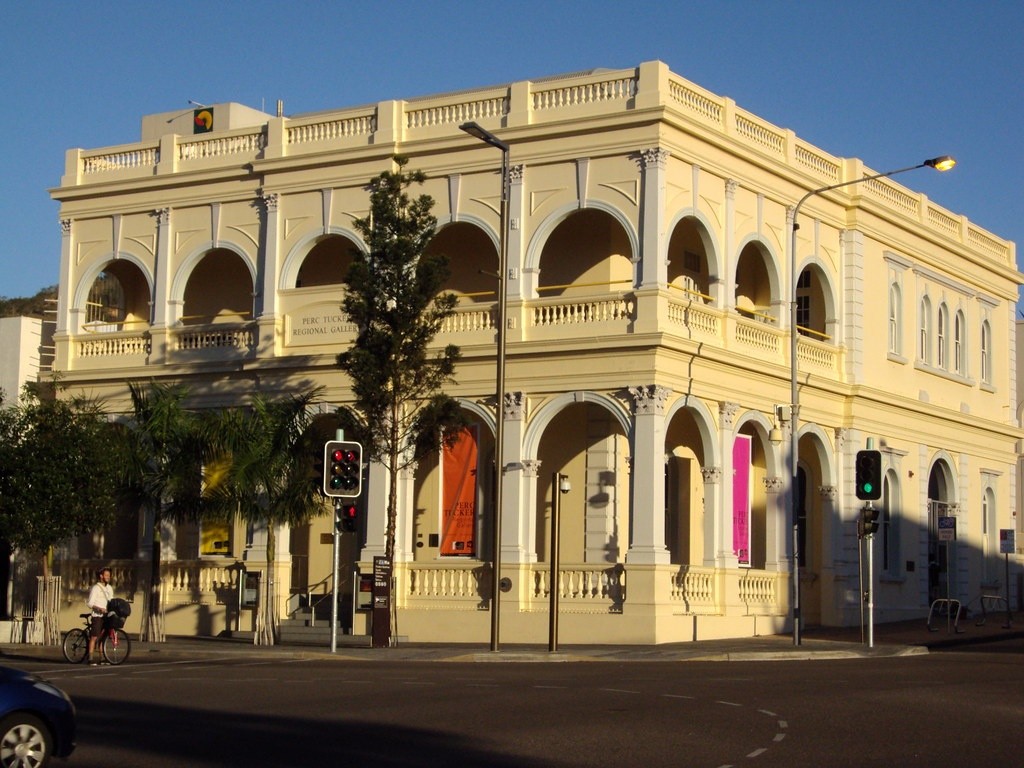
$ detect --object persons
[87,567,114,667]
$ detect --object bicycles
[63,612,131,665]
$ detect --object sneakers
[100,659,112,665]
[88,659,97,665]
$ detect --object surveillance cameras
[561,482,571,494]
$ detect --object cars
[0,665,77,768]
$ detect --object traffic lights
[338,501,360,533]
[323,441,363,498]
[860,508,879,536]
[854,448,882,502]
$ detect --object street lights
[462,119,511,653]
[791,155,957,644]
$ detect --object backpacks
[109,597,131,617]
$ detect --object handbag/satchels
[107,612,125,627]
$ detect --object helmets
[95,566,111,573]
